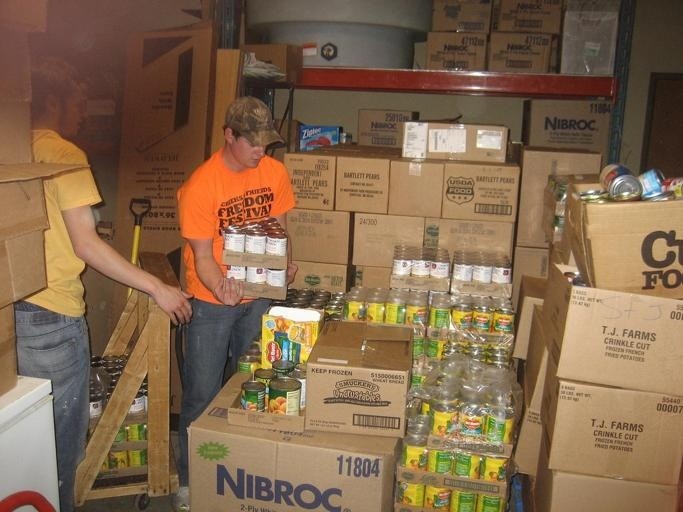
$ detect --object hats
[224,96,286,149]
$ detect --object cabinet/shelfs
[297,67,630,102]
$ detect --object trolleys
[71,253,180,509]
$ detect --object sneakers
[174,485,191,512]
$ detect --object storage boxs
[285,98,521,294]
[0,0,90,398]
[188,27,218,181]
[426,2,615,74]
[509,99,680,507]
[187,321,415,509]
[239,42,302,85]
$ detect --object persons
[13,58,195,512]
[172,97,298,509]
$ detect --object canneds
[339,132,352,146]
[237,246,517,512]
[85,353,149,472]
[547,163,683,285]
[226,265,286,288]
[224,218,287,256]
